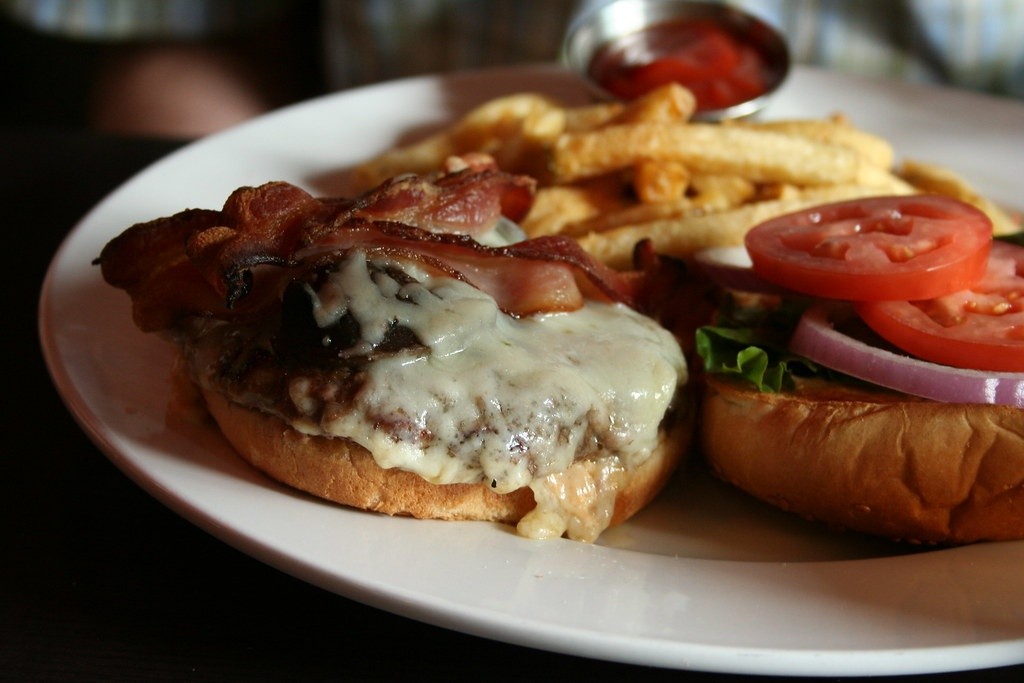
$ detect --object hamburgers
[89,167,1024,547]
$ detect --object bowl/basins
[564,0,794,125]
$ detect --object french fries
[348,85,1024,261]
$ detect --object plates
[41,62,1024,674]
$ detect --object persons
[16,0,1023,139]
